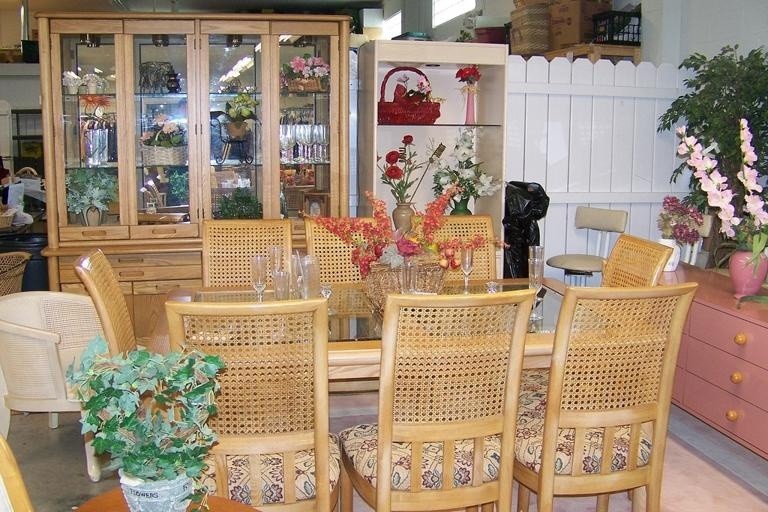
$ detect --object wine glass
[528,246,544,304]
[461,248,473,294]
[279,124,330,164]
[248,247,321,302]
[528,258,544,321]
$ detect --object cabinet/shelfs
[658,261,768,460]
[32,14,355,343]
[356,39,509,292]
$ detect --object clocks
[300,193,327,221]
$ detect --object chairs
[139,171,293,289]
[0,285,114,480]
[0,252,33,298]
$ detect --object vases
[391,201,414,229]
[450,194,471,214]
[464,81,478,125]
[726,253,767,296]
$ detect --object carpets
[315,370,766,512]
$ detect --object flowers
[375,130,437,200]
[671,125,767,245]
[138,115,191,147]
[279,49,329,87]
[454,65,485,81]
[423,127,497,199]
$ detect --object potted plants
[64,168,116,227]
[219,92,261,140]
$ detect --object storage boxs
[546,0,611,51]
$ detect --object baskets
[0,200,16,229]
[362,261,447,317]
[284,71,329,95]
[140,128,189,166]
[376,66,442,126]
